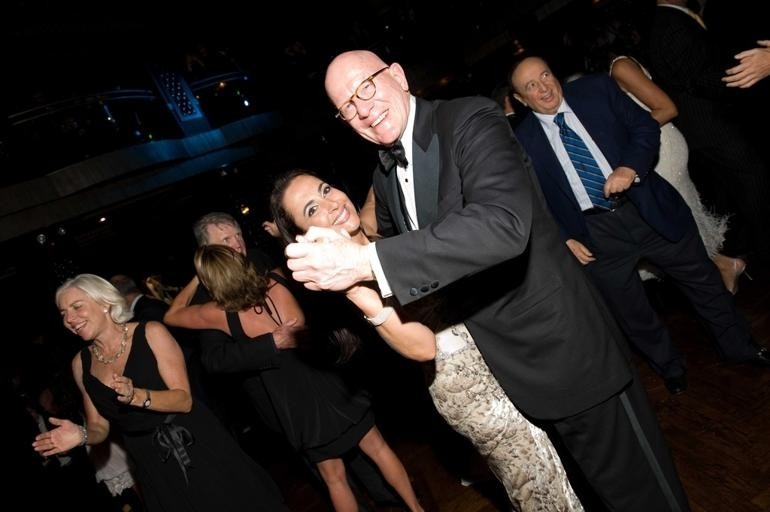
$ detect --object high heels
[727,258,752,294]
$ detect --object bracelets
[362,307,392,327]
[77,424,88,445]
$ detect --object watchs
[142,389,152,409]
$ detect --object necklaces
[91,323,128,364]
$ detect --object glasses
[334,67,390,121]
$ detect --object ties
[553,112,616,209]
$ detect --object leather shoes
[724,345,770,373]
[664,356,689,394]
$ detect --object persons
[638,1,709,83]
[286,49,691,512]
[31,273,289,512]
[721,40,770,91]
[163,244,425,511]
[191,212,321,420]
[562,39,753,296]
[501,52,770,395]
[1,214,282,506]
[271,170,584,512]
[481,69,519,130]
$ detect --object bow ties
[377,140,409,172]
